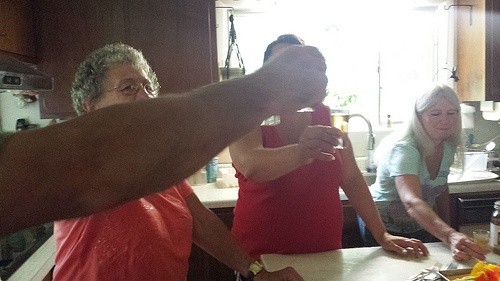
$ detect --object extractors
[0,55,55,91]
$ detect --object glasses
[91,80,162,96]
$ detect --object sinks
[362,175,377,185]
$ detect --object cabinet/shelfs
[0,0,219,119]
[452,0,500,102]
[189,209,236,281]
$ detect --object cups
[187,156,219,186]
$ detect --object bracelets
[247,260,264,281]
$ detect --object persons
[0,45,327,237]
[228,34,430,261]
[53,42,305,281]
[357,85,488,261]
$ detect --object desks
[261,242,500,281]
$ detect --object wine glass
[473,230,491,264]
[329,108,350,149]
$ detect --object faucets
[349,113,377,172]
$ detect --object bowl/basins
[462,152,488,172]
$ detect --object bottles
[490,201,500,255]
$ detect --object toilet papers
[481,102,500,121]
